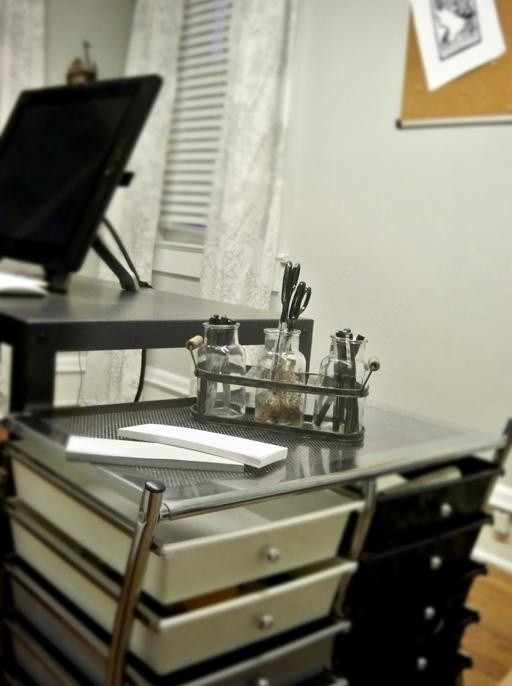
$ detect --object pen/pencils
[200,314,236,415]
[315,328,365,434]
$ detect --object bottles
[197,320,368,434]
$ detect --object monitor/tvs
[0,73,164,294]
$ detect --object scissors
[275,261,311,366]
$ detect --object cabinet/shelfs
[4,391,509,685]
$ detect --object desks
[1,269,316,414]
